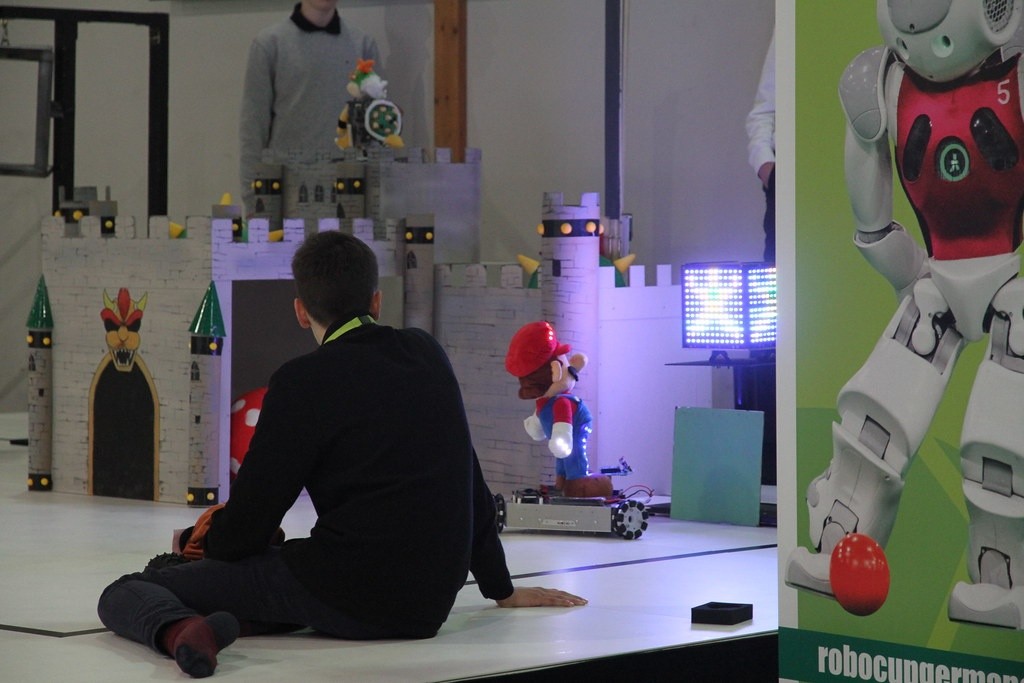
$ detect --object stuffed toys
[334,59,405,148]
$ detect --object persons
[784,0,1024,629]
[98,232,589,678]
[238,0,383,154]
[746,29,776,262]
[505,321,613,497]
